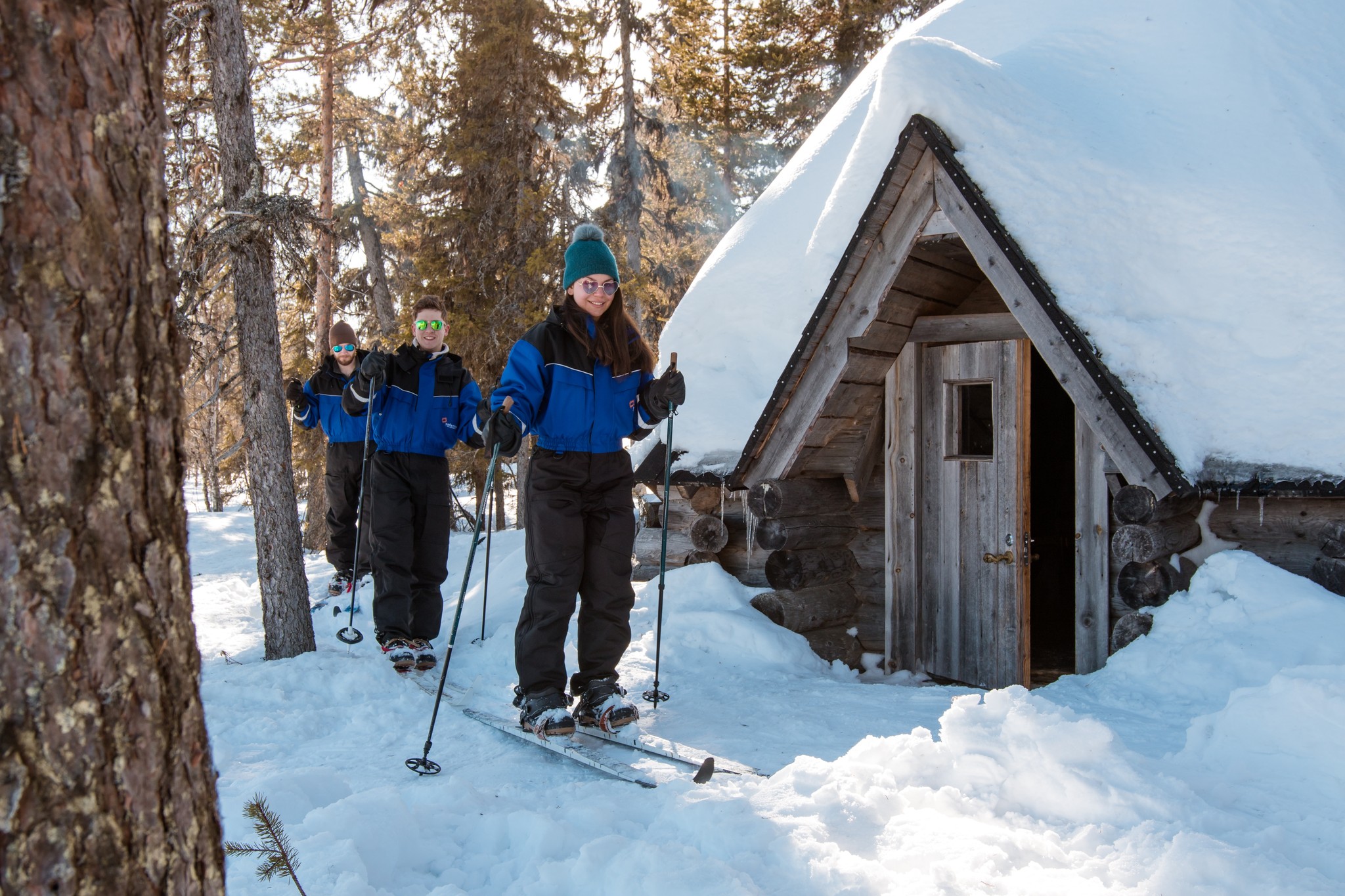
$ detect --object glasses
[575,280,620,296]
[413,320,446,331]
[332,344,355,352]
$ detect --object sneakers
[382,637,421,668]
[581,677,637,726]
[327,574,353,595]
[408,639,437,667]
[360,574,372,586]
[523,687,576,734]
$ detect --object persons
[482,224,685,735]
[341,295,496,667]
[285,320,376,596]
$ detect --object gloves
[660,369,686,406]
[489,411,517,453]
[361,350,387,380]
[286,377,304,401]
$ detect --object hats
[329,320,357,349]
[562,223,620,290]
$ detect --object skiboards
[374,643,483,706]
[310,583,359,616]
[460,698,766,788]
